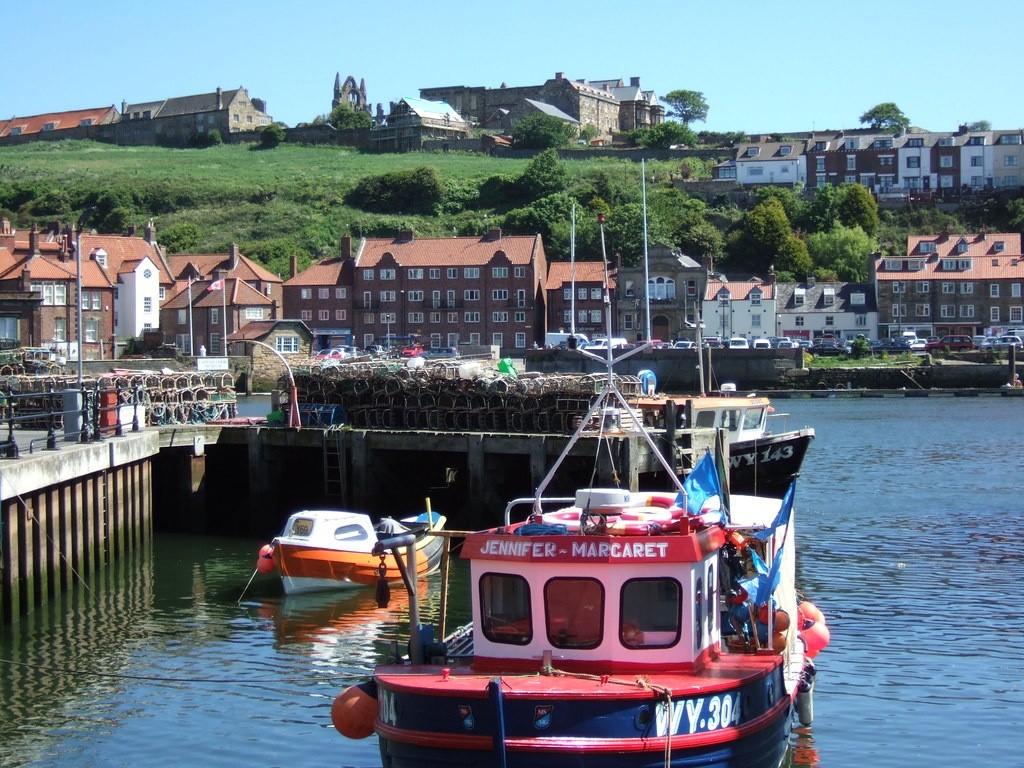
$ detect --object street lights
[76,206,95,389]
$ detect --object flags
[736,545,783,607]
[743,482,796,542]
[673,452,721,515]
[207,279,225,292]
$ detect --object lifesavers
[543,496,721,534]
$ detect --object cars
[311,344,401,363]
[544,328,1024,354]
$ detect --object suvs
[419,347,459,358]
[401,342,425,358]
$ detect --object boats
[373,157,818,768]
[270,496,451,596]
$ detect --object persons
[531,341,539,349]
[200,344,207,357]
[548,342,554,348]
